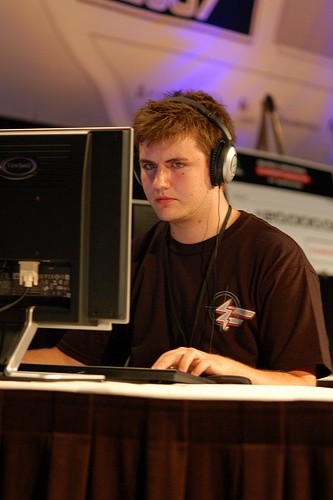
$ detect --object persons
[20,86,333,386]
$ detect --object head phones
[134,96,238,186]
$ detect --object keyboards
[0,364,216,384]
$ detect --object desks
[0,380,332,500]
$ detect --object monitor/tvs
[0,126,135,331]
